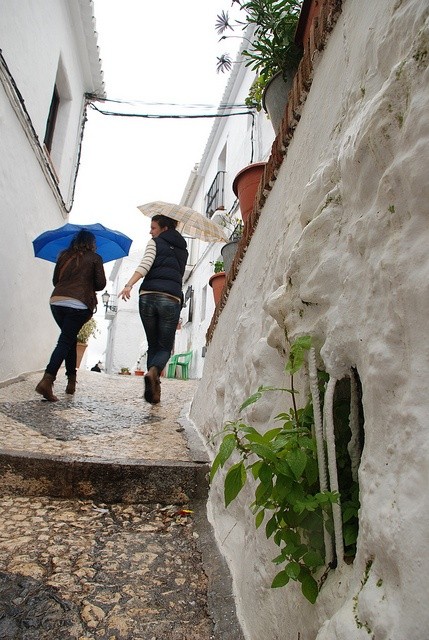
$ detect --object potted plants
[208,260,226,307]
[118,367,131,375]
[217,211,244,277]
[244,66,294,137]
[134,349,148,376]
[75,317,101,369]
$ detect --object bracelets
[124,284,133,289]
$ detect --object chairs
[167,352,192,379]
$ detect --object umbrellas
[137,198,229,245]
[31,222,133,264]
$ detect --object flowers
[214,0,302,74]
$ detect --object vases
[232,162,269,224]
[285,45,302,69]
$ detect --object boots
[66,376,76,394]
[145,367,158,402]
[35,372,57,402]
[152,376,161,403]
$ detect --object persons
[91,363,101,372]
[36,231,106,402]
[116,212,188,404]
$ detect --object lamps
[102,290,117,312]
